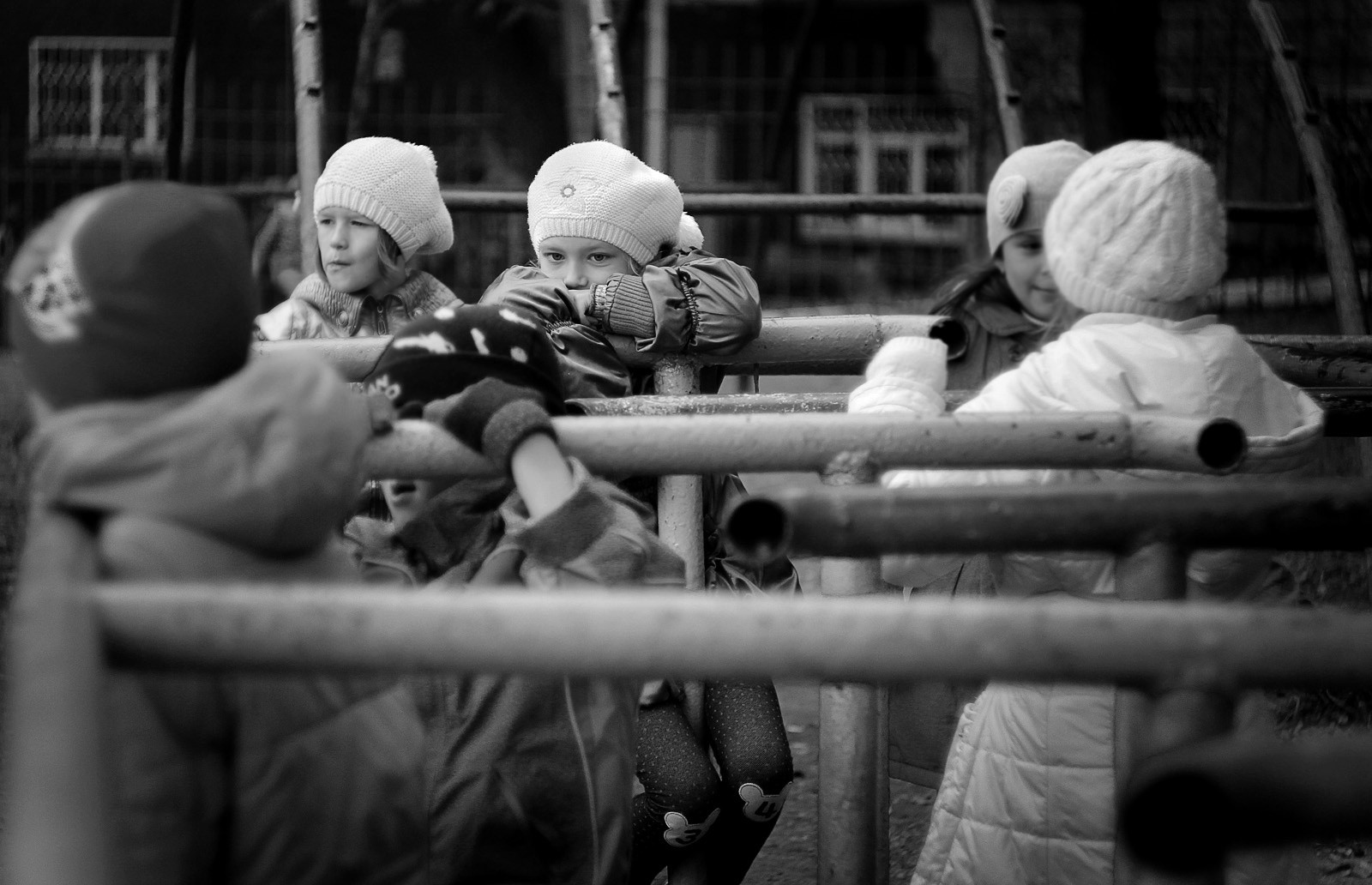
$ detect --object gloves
[865,336,949,395]
[364,392,397,434]
[422,378,561,468]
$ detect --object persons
[0,177,439,885]
[849,136,1321,885]
[248,136,798,885]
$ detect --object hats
[1043,141,1229,319]
[5,182,256,411]
[313,136,454,262]
[986,139,1096,256]
[360,304,567,416]
[528,140,707,266]
